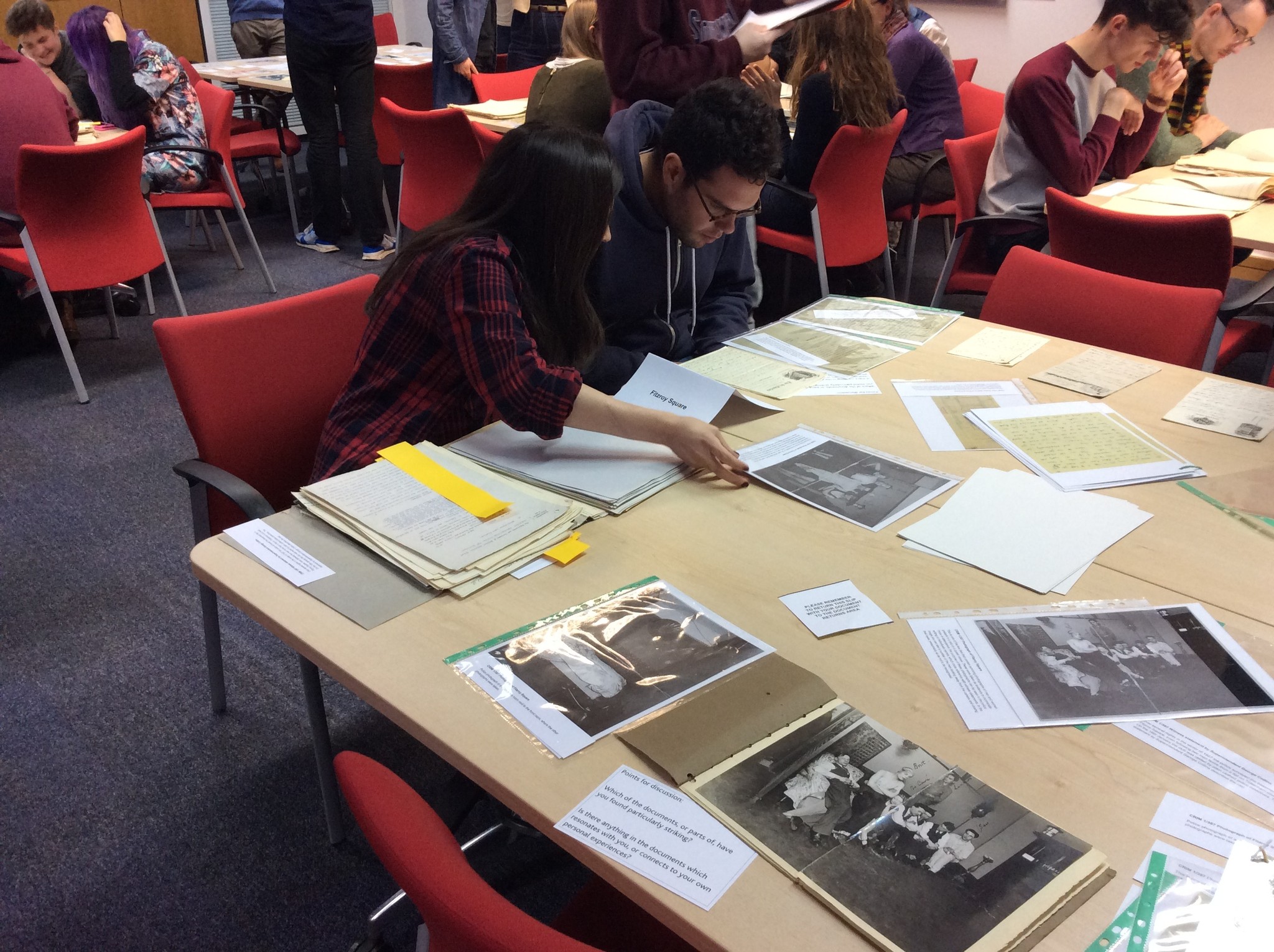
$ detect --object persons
[5,0,103,121]
[1113,0,1274,172]
[741,0,906,296]
[226,0,293,127]
[907,5,955,73]
[974,0,1196,275]
[863,0,965,288]
[1036,619,1181,695]
[779,447,892,510]
[428,0,498,108]
[282,0,395,260]
[596,0,794,113]
[506,0,577,72]
[525,0,611,128]
[495,0,513,54]
[309,121,751,487]
[584,78,783,389]
[0,39,79,346]
[791,753,979,875]
[67,6,208,317]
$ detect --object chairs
[155,274,386,844]
[0,56,300,405]
[330,750,697,952]
[755,58,1273,386]
[336,12,546,256]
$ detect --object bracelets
[1147,94,1172,107]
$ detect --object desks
[430,83,795,139]
[187,293,1274,952]
[191,43,433,219]
[1043,162,1273,254]
[74,114,130,300]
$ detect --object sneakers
[295,223,340,252]
[361,235,397,260]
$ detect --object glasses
[687,165,763,223]
[1222,8,1255,48]
